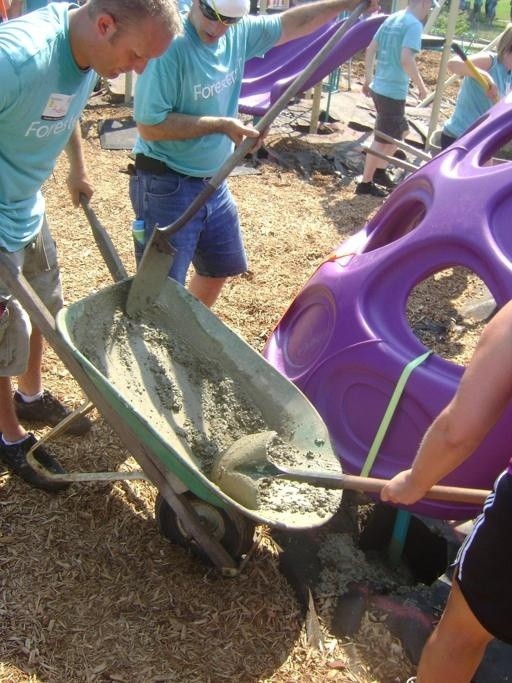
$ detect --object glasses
[197,1,244,26]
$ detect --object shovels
[207,431,494,510]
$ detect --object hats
[204,1,252,19]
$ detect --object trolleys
[0,193,345,580]
[348,118,512,173]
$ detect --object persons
[0,0,185,493]
[356,0,436,197]
[379,296,512,683]
[8,0,46,21]
[441,23,512,165]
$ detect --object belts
[134,151,213,182]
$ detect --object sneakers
[12,389,92,439]
[373,167,399,189]
[353,181,388,197]
[0,432,73,495]
